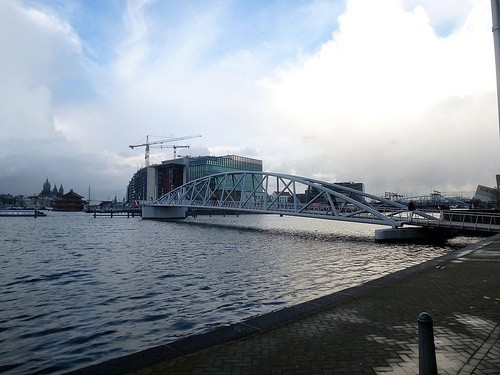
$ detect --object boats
[0,209,47,218]
[375,227,460,243]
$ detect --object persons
[408,200,416,221]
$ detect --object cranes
[129,134,202,166]
[150,144,190,159]
